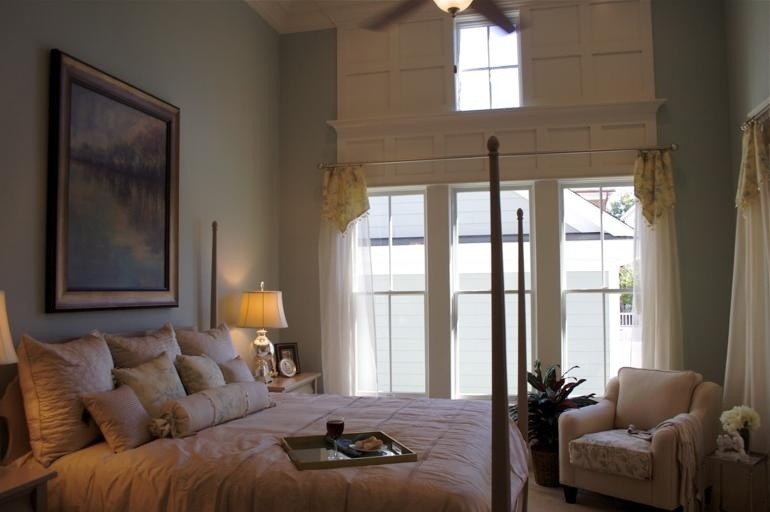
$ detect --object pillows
[112,350,189,421]
[173,320,236,365]
[15,329,119,469]
[78,384,160,455]
[148,381,278,440]
[102,322,186,367]
[172,352,226,395]
[219,355,257,385]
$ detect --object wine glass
[326,414,345,461]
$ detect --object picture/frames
[43,46,182,315]
[274,343,301,377]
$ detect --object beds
[1,135,537,512]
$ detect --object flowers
[719,406,761,434]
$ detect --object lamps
[433,1,474,17]
[236,281,289,383]
[2,290,21,465]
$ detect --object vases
[736,428,751,456]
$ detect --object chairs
[557,366,725,511]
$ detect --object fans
[364,1,515,34]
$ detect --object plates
[349,444,387,452]
[280,357,297,377]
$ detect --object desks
[699,449,769,511]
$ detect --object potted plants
[508,360,600,489]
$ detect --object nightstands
[252,371,323,395]
[1,464,60,512]
